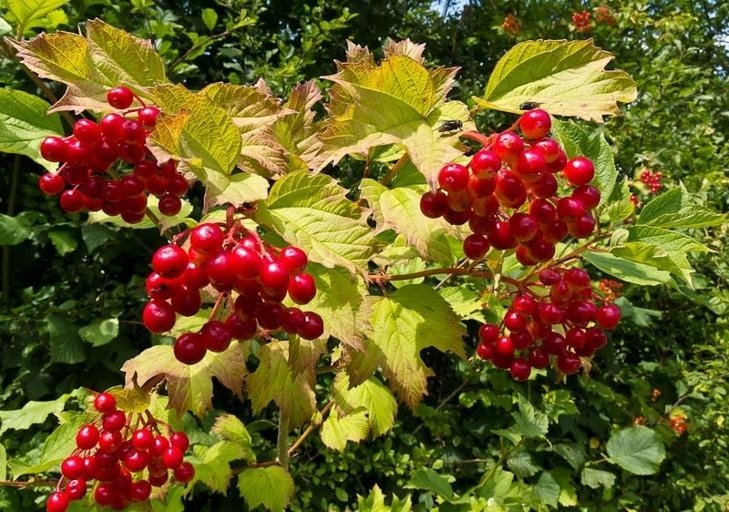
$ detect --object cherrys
[38,6,687,512]
